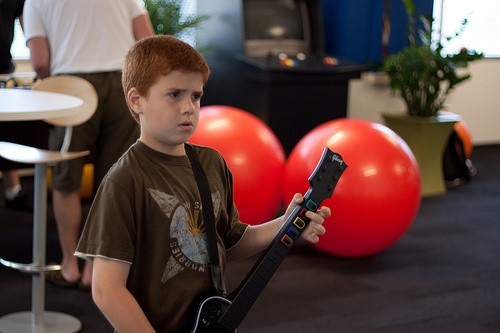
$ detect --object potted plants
[380,15,485,198]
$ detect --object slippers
[50,269,80,287]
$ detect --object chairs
[0,76,98,332]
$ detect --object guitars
[175,146,348,333]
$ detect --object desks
[1,88,84,121]
[233,53,363,161]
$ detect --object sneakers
[4,186,35,213]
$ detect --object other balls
[187,104,286,225]
[282,118,421,257]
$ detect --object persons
[0,0,157,288]
[73,35,331,333]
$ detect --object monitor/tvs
[243,0,311,59]
